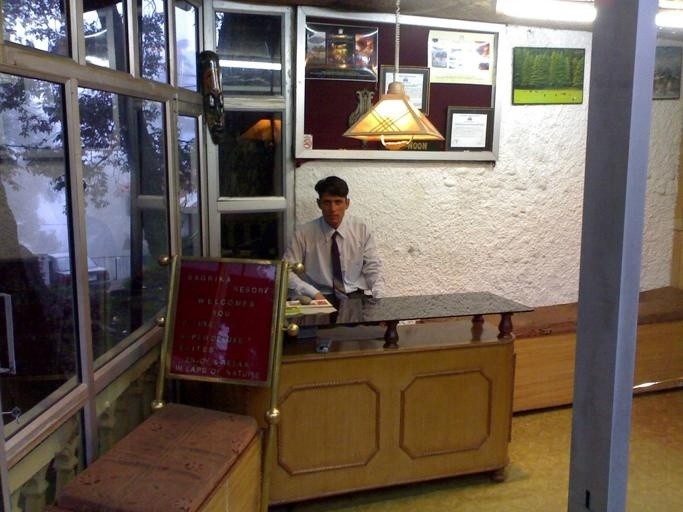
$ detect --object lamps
[341,2,447,153]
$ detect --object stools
[50,399,264,512]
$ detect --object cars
[47,252,111,294]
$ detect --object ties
[332,232,347,299]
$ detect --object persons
[281,175,383,302]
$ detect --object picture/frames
[652,45,683,101]
[378,61,432,118]
[443,105,495,152]
